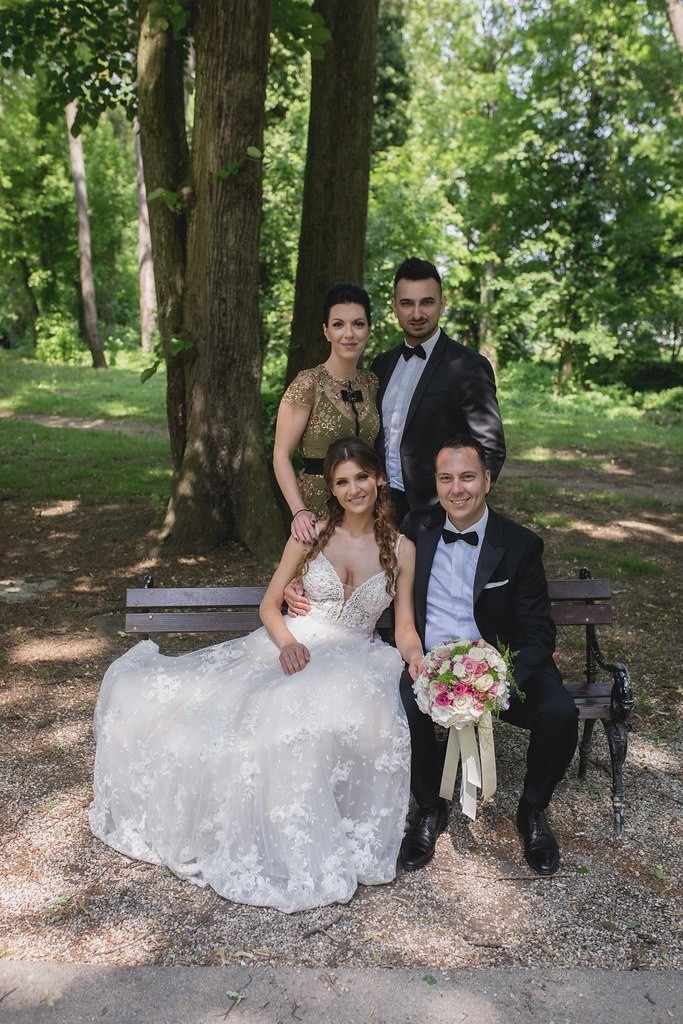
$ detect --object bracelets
[293,509,311,520]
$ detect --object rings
[284,657,290,662]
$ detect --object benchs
[123,565,633,838]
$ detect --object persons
[272,282,381,545]
[371,256,507,638]
[279,434,580,876]
[242,437,430,882]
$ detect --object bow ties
[401,344,427,362]
[441,528,479,547]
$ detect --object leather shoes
[400,802,448,870]
[516,806,559,875]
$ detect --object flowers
[410,637,528,732]
